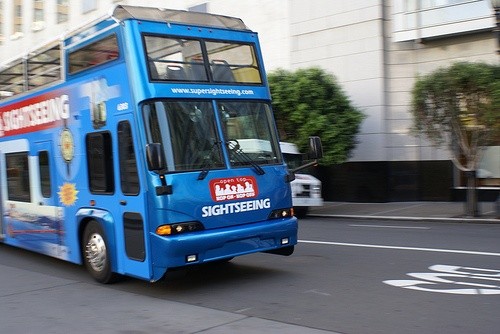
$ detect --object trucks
[228,140,323,219]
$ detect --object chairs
[147,57,239,167]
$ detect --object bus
[2,6,324,286]
